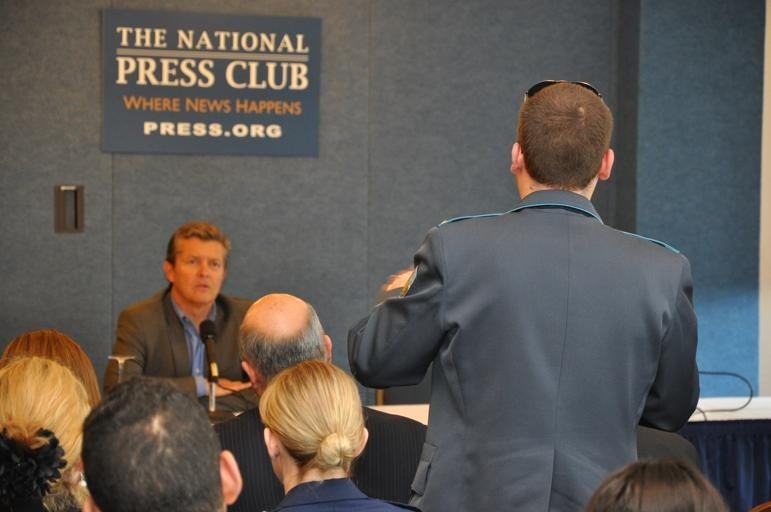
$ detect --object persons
[0,358,93,510]
[258,361,421,512]
[349,77,697,512]
[585,458,730,512]
[212,293,426,509]
[0,328,104,409]
[103,221,261,414]
[80,378,244,510]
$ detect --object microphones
[199,320,220,384]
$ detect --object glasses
[523,79,602,101]
